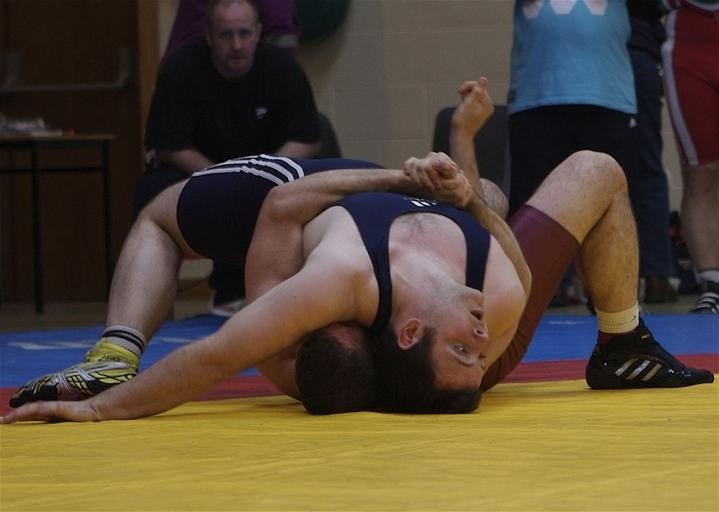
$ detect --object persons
[1,75,531,425]
[657,1,717,312]
[245,149,712,415]
[158,3,302,311]
[546,1,682,306]
[130,1,324,225]
[506,1,639,315]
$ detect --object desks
[1,126,114,318]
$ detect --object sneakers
[8,339,140,409]
[688,279,719,315]
[585,319,714,392]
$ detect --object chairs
[429,101,510,187]
[318,115,341,159]
